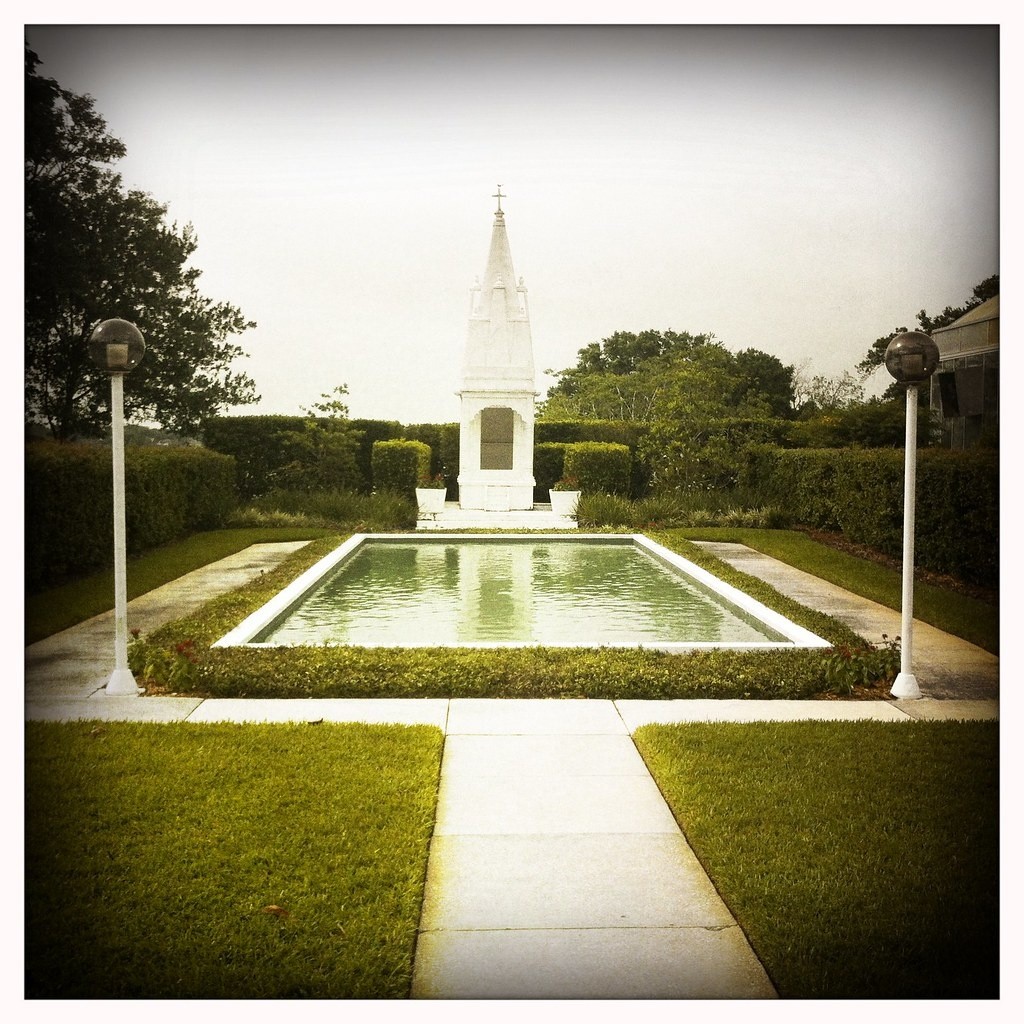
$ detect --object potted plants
[550,479,582,515]
[415,473,448,514]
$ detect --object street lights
[90,318,146,694]
[885,332,938,697]
